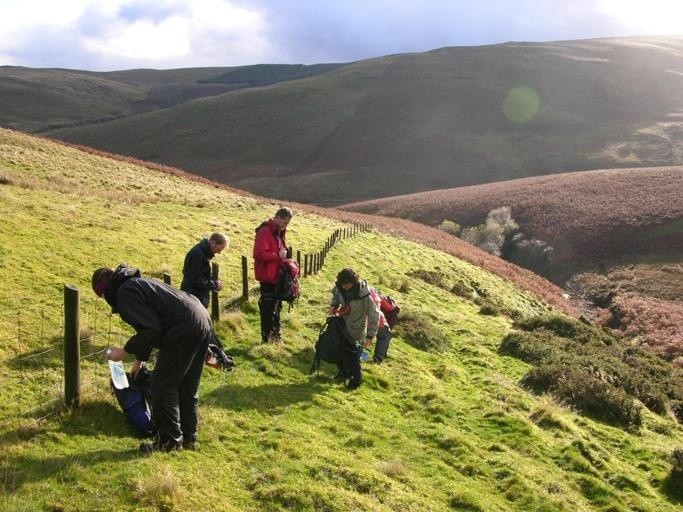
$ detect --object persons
[180,232,228,310]
[328,267,379,390]
[368,286,400,364]
[92,262,212,453]
[253,208,293,345]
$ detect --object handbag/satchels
[315,316,358,364]
[275,258,300,302]
[109,368,156,431]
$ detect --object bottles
[106,349,130,390]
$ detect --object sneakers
[140,436,196,452]
[334,371,360,389]
